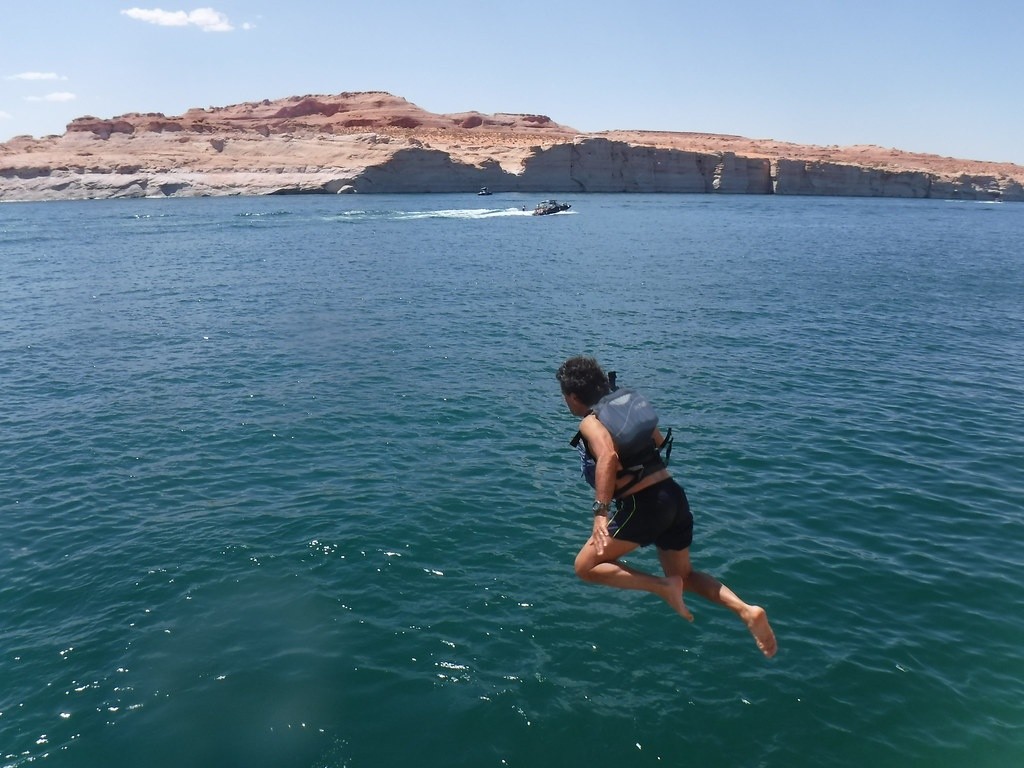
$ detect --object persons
[555,357,778,657]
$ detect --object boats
[532,199,571,216]
[478,186,492,195]
[995,198,1003,202]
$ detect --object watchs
[592,500,611,512]
